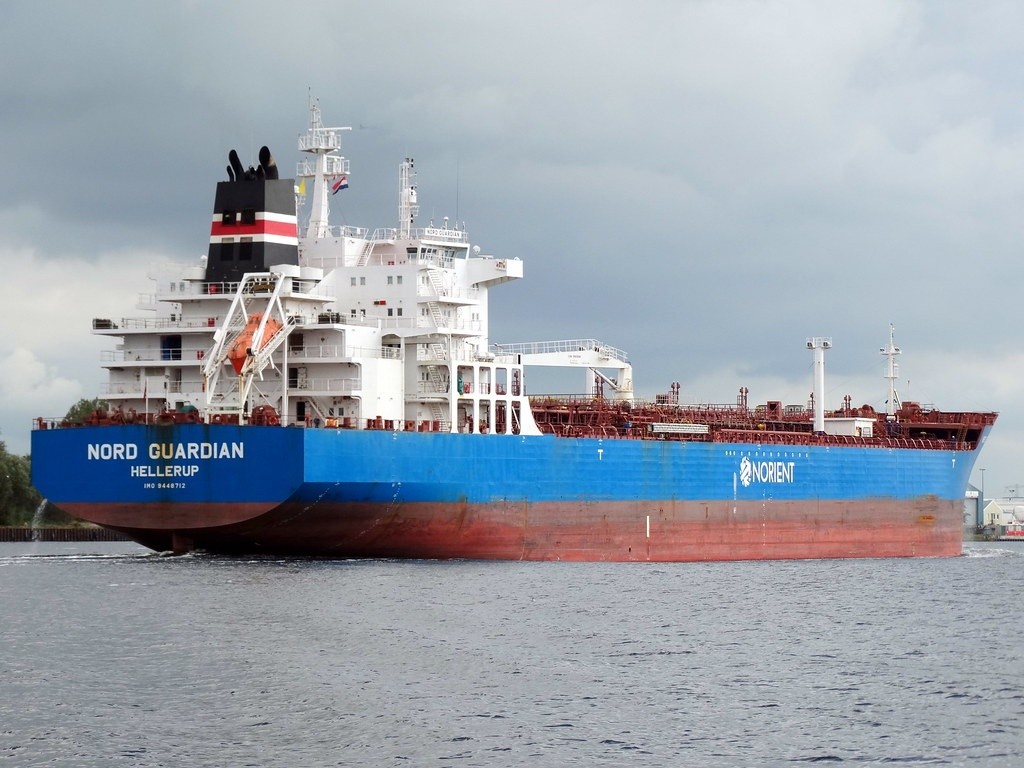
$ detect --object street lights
[979,469,986,493]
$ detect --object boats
[29,85,1001,562]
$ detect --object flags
[332,176,348,195]
[299,178,308,196]
